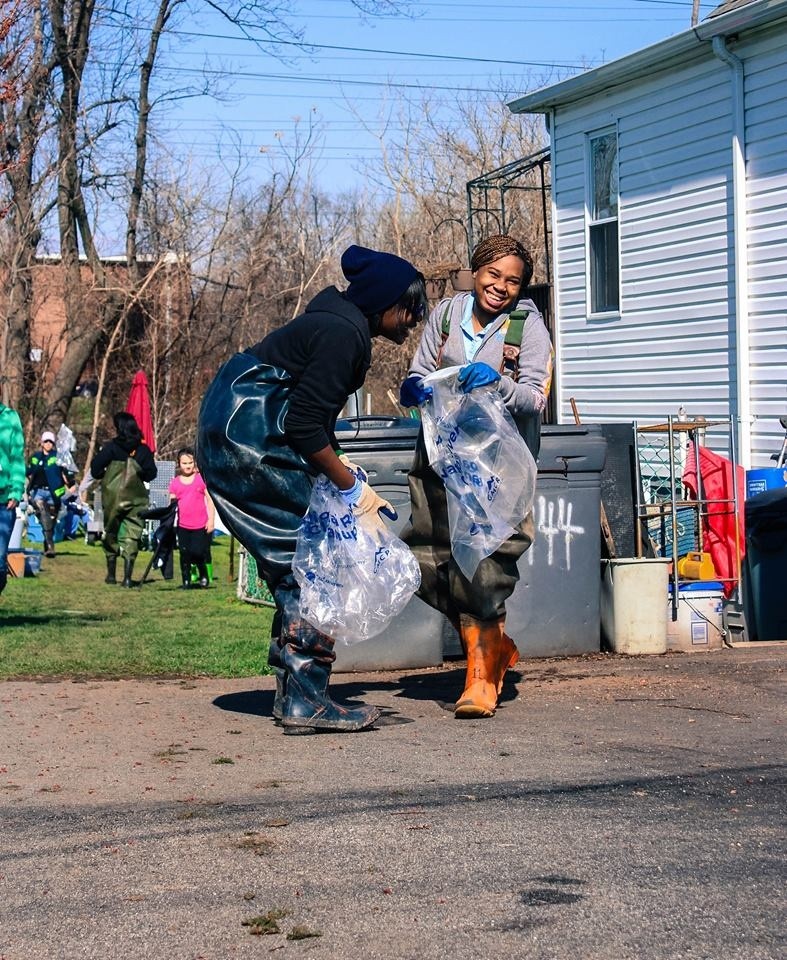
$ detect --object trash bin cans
[326,415,444,673]
[744,488,787,641]
[444,423,609,660]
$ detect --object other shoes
[183,579,190,588]
[200,577,209,589]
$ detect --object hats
[341,246,418,315]
[41,432,56,444]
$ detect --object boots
[105,560,116,583]
[453,613,506,716]
[497,632,520,695]
[43,531,55,556]
[268,635,288,727]
[280,625,382,735]
[121,558,134,588]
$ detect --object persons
[0,386,26,596]
[54,423,80,487]
[89,411,158,587]
[393,232,556,721]
[197,244,432,738]
[167,447,215,591]
[25,430,70,559]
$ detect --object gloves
[457,361,502,393]
[338,454,369,484]
[399,377,433,409]
[339,475,398,548]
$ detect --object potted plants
[421,260,461,297]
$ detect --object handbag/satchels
[415,364,539,584]
[290,471,422,648]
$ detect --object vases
[447,266,481,292]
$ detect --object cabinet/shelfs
[631,415,746,612]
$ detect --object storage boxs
[26,508,66,524]
[26,521,66,543]
